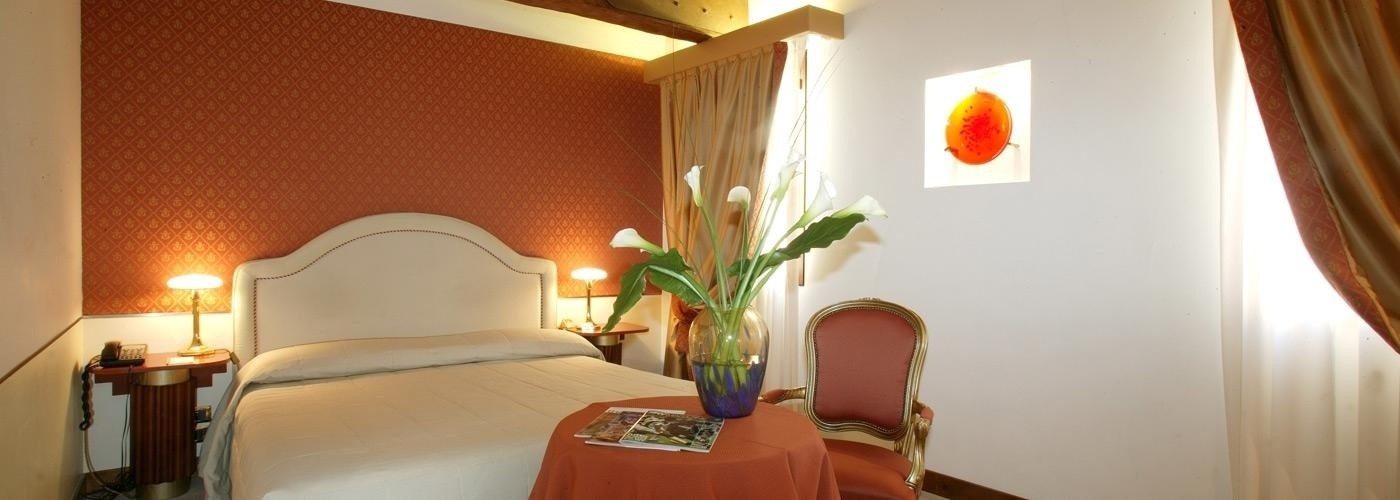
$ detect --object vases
[688,304,770,420]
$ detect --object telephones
[99,339,147,366]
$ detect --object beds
[194,211,699,500]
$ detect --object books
[573,406,623,439]
[586,410,686,451]
[618,409,726,453]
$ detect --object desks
[527,395,842,500]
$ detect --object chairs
[758,297,935,500]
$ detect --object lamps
[166,274,224,356]
[571,267,608,330]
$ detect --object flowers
[601,153,888,335]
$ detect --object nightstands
[559,321,649,365]
[89,348,232,500]
